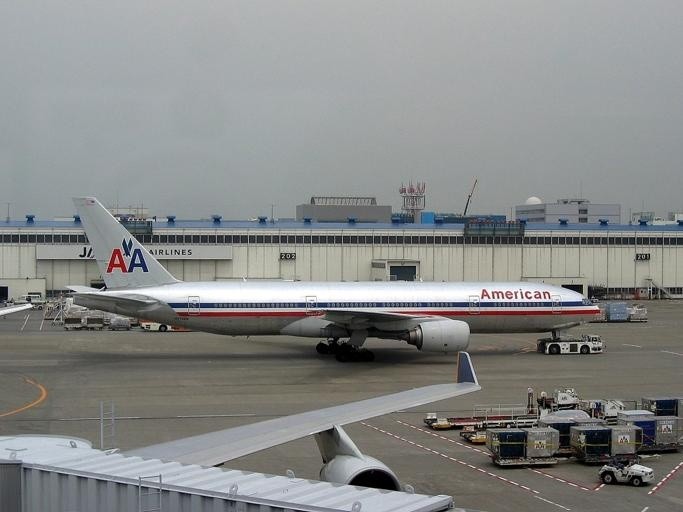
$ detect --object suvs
[598,461,653,486]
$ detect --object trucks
[537,334,605,354]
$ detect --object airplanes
[0,352,482,490]
[65,197,600,362]
